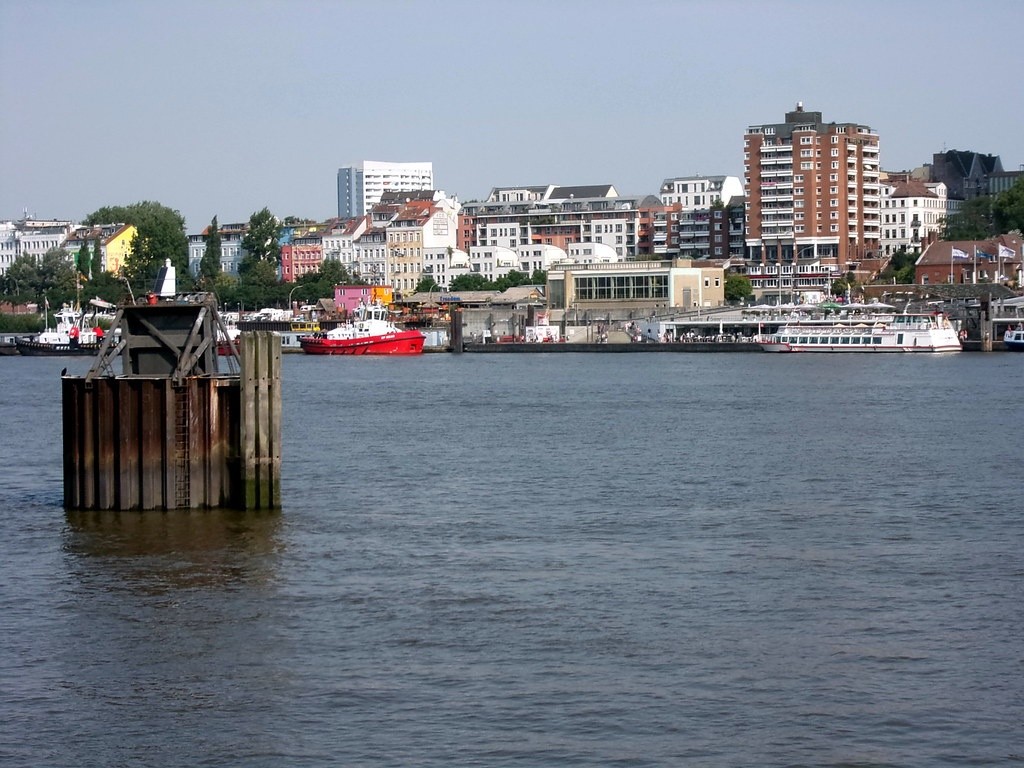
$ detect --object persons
[663,331,756,343]
[596,320,662,344]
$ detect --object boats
[0,272,124,356]
[297,296,425,356]
[752,312,963,353]
[1003,328,1024,351]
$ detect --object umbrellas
[746,302,896,317]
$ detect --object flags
[78,273,87,289]
[999,244,1016,258]
[952,246,970,259]
[46,299,50,309]
[975,246,992,258]
